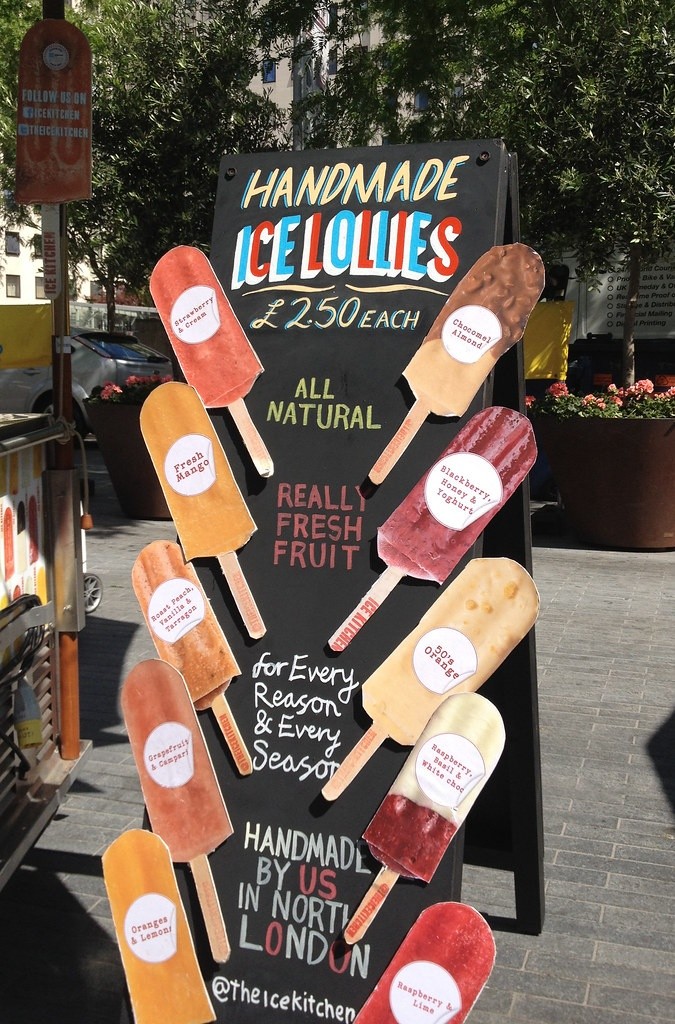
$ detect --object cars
[0,322,177,451]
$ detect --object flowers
[526,379,675,418]
[89,374,174,402]
